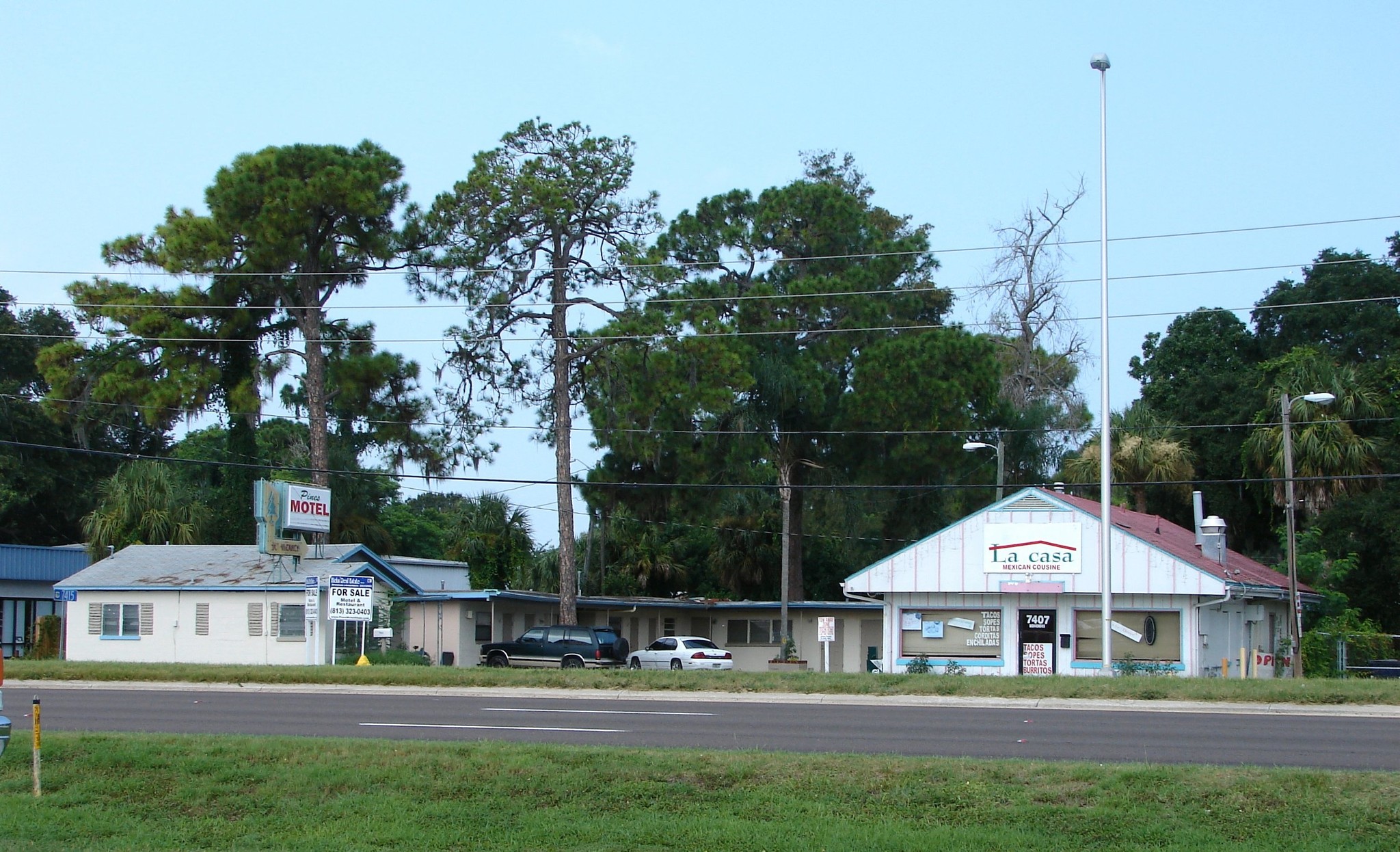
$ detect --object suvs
[476,624,630,670]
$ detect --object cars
[626,636,733,671]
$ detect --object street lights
[962,441,1006,502]
[1281,389,1337,678]
[1088,50,1117,678]
